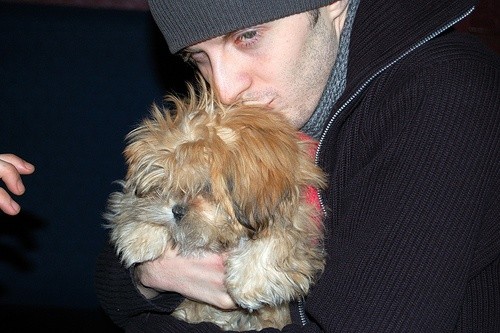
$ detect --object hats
[147,0,339,54]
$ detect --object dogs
[96,70,334,332]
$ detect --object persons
[98,0,500,333]
[1,0,191,333]
